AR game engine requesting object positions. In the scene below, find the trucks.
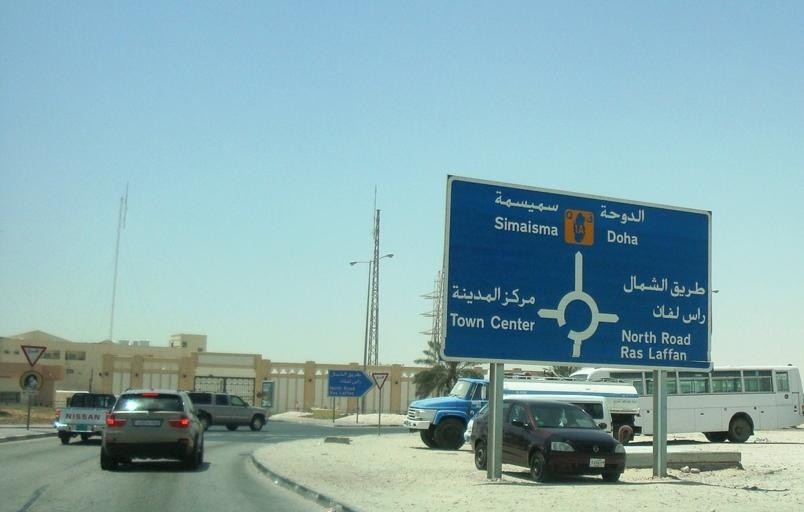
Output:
[404,378,642,450]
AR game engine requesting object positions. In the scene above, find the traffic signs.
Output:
[328,367,378,399]
[438,172,717,373]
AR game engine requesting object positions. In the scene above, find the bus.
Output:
[568,366,803,445]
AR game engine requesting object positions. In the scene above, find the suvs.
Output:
[98,386,205,472]
[186,391,269,432]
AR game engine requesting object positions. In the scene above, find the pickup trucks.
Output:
[54,389,118,447]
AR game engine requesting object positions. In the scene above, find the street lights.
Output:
[346,253,395,413]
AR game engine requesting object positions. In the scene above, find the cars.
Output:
[465,395,627,485]
